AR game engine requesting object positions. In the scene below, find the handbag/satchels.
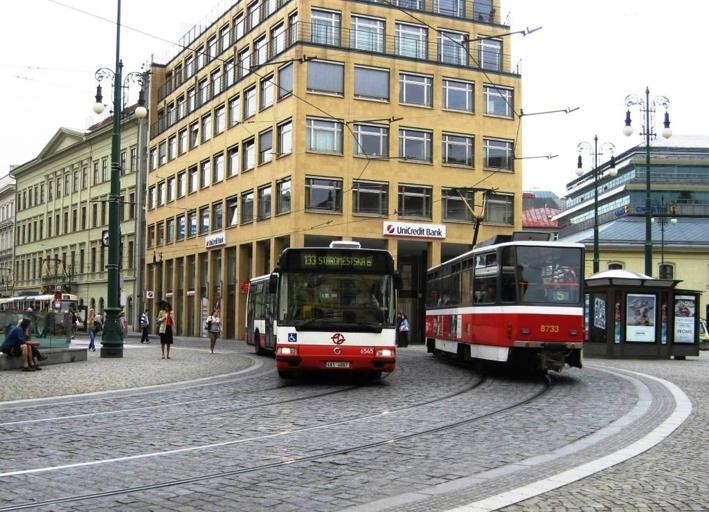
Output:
[204,314,214,331]
[399,325,409,333]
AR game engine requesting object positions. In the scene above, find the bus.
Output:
[1,293,80,338]
[423,229,584,379]
[232,230,404,382]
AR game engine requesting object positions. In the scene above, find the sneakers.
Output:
[89,346,91,351]
[93,348,96,352]
[37,355,50,361]
[141,339,152,344]
[29,365,42,370]
[161,354,166,359]
[167,355,171,359]
[209,351,216,354]
[21,366,36,372]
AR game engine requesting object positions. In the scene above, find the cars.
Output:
[699,318,709,342]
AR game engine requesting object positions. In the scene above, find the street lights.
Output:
[91,59,149,359]
[651,194,677,280]
[63,264,77,292]
[575,134,618,273]
[623,84,673,278]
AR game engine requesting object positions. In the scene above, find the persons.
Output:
[397,313,409,348]
[437,254,577,308]
[157,303,175,359]
[16,318,48,361]
[139,307,151,345]
[94,312,103,328]
[0,316,43,372]
[120,311,129,344]
[26,306,79,345]
[348,276,381,308]
[395,311,402,345]
[205,308,222,355]
[86,308,97,353]
